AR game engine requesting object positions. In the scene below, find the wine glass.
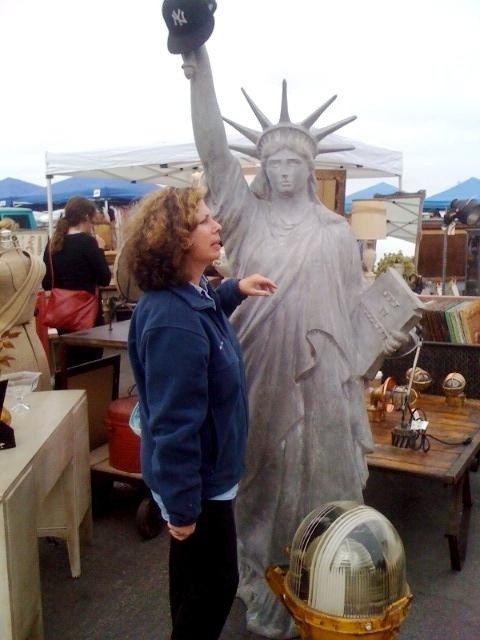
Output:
[1,370,44,417]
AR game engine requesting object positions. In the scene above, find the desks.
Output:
[364,385,480,571]
[61,319,165,538]
[0,388,93,640]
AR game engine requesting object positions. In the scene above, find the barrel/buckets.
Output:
[104,395,140,472]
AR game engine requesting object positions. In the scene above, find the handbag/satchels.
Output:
[42,287,99,330]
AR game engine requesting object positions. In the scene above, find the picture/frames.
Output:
[56,352,122,452]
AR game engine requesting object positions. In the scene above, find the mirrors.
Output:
[350,190,425,283]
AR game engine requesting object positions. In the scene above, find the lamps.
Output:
[440,198,480,295]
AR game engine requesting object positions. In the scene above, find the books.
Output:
[349,266,428,383]
[418,299,479,346]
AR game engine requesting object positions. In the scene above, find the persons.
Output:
[120,182,280,640]
[178,40,414,639]
[40,194,112,371]
